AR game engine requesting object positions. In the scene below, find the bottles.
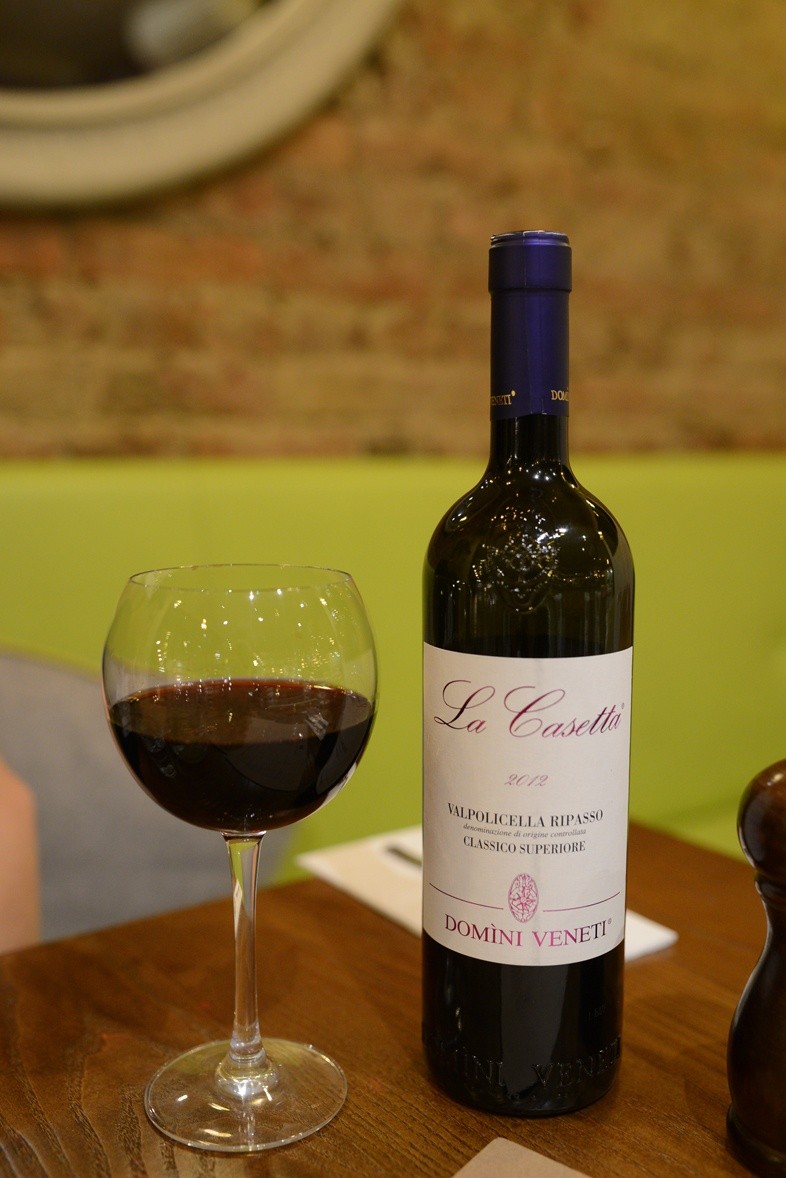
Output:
[723,759,786,1178]
[421,226,636,1121]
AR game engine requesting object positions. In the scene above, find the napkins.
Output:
[293,830,680,975]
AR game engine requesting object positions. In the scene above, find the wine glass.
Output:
[100,562,377,1153]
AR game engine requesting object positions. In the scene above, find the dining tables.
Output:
[2,822,774,1178]
